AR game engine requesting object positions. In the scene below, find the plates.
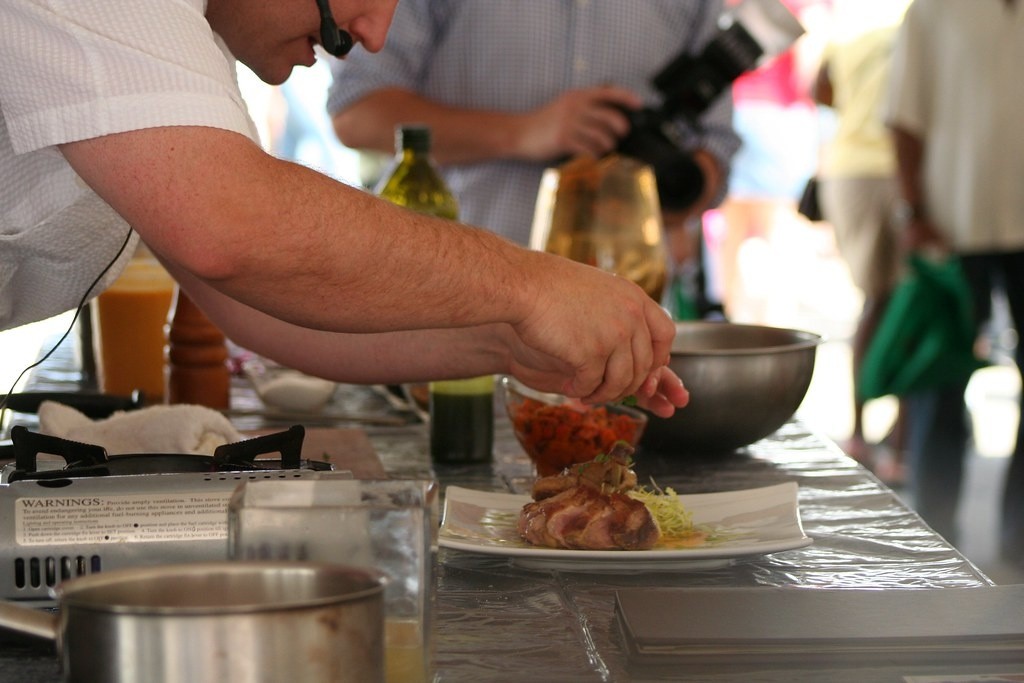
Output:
[439,481,812,571]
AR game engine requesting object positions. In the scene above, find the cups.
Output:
[431,378,496,465]
[92,236,180,394]
[534,165,667,302]
[228,469,438,682]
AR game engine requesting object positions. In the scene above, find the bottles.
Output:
[380,125,461,226]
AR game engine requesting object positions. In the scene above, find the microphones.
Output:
[316,0,353,55]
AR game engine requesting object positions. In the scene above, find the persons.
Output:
[328,0,739,324]
[0,0,690,417]
[813,0,1024,560]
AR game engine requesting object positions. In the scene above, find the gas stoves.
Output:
[0,425,376,602]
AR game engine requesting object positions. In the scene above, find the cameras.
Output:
[604,1,804,213]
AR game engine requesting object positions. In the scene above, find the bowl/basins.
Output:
[635,319,823,455]
[50,561,388,683]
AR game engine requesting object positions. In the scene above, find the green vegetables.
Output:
[579,390,639,475]
